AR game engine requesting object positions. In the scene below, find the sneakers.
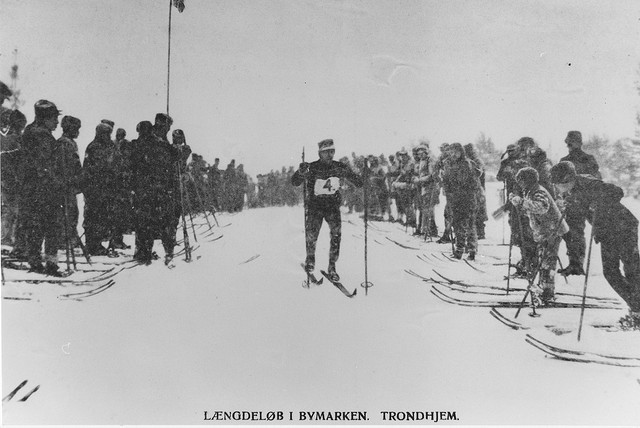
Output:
[328,266,340,282]
[536,291,556,301]
[562,263,585,275]
[305,257,315,272]
[621,316,639,329]
[29,261,45,273]
[469,251,476,259]
[454,251,463,261]
[44,263,62,277]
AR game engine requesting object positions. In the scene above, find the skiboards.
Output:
[424,269,623,301]
[0,266,125,284]
[174,212,239,245]
[343,219,426,250]
[487,307,620,333]
[300,262,357,298]
[2,279,116,300]
[57,257,141,269]
[428,286,624,307]
[419,242,512,271]
[525,332,640,366]
[110,246,200,259]
[402,269,462,284]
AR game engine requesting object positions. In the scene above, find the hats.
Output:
[550,160,576,184]
[61,115,81,129]
[35,99,62,117]
[515,166,539,185]
[10,108,26,125]
[449,142,466,154]
[416,144,429,155]
[155,113,173,125]
[95,123,112,133]
[318,137,336,152]
[564,130,582,147]
[137,120,153,131]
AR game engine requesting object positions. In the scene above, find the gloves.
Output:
[361,166,370,177]
[299,161,311,175]
[512,195,522,206]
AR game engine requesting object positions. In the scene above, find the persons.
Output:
[85,124,115,256]
[152,113,173,141]
[557,130,602,275]
[464,143,488,239]
[111,128,133,235]
[10,101,62,261]
[193,153,300,212]
[128,119,192,264]
[100,119,115,124]
[508,167,570,306]
[548,161,640,331]
[291,139,363,281]
[339,142,449,244]
[445,142,481,260]
[26,104,67,278]
[518,136,535,153]
[56,114,85,250]
[170,128,193,216]
[1,81,24,245]
[494,143,529,244]
[526,146,556,200]
